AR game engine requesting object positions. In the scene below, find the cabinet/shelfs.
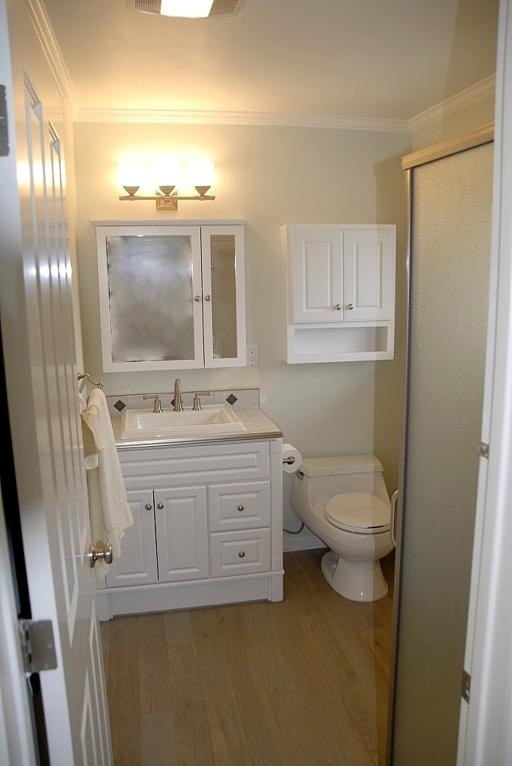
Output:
[207,479,275,579]
[96,223,246,375]
[100,485,210,589]
[280,220,400,366]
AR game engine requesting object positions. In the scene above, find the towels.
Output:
[81,388,135,582]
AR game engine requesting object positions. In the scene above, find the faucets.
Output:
[174,378,185,411]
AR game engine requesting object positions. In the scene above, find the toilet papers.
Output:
[282,441,303,475]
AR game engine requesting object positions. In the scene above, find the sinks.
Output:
[121,403,248,439]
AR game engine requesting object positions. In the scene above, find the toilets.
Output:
[293,455,395,603]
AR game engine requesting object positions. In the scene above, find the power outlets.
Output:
[246,344,259,367]
